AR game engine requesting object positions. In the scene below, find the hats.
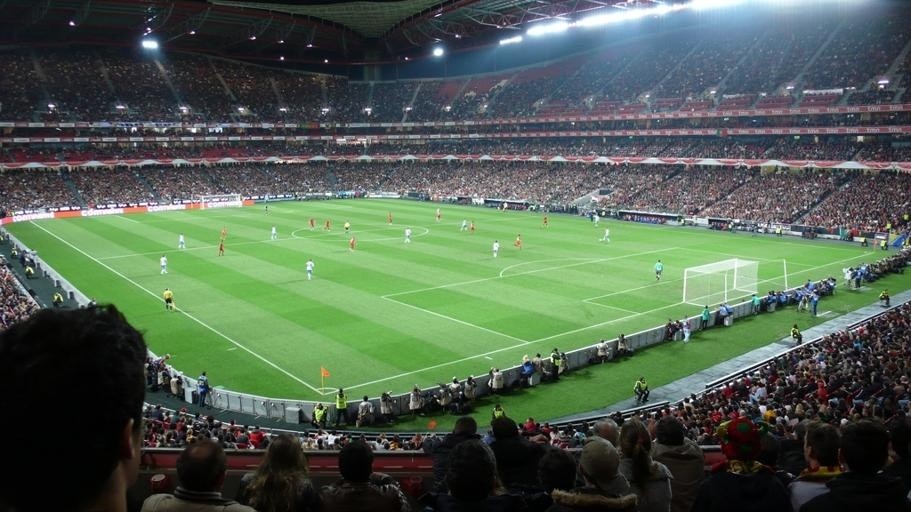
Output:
[579,438,632,497]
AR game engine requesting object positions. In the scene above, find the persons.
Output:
[0,11,910,512]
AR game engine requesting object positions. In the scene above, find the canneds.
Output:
[149,474,168,492]
